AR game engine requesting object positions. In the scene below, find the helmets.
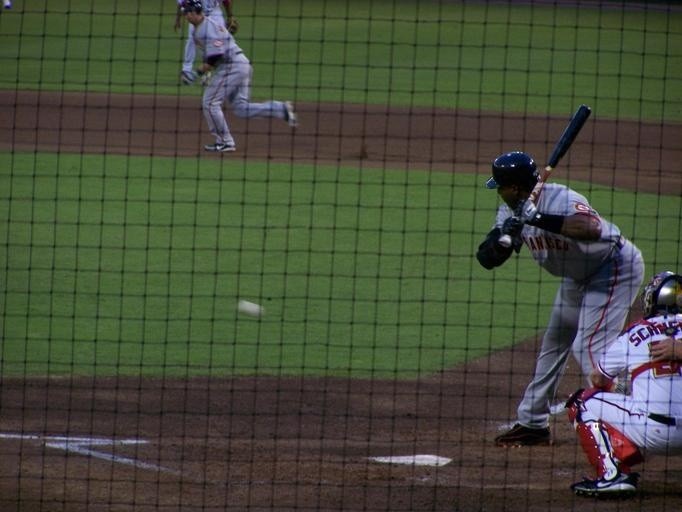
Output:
[181,0,203,16]
[641,271,682,319]
[486,152,536,189]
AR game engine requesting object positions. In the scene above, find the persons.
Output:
[649,337,682,362]
[475,149,647,441]
[3,0,11,9]
[172,0,239,83]
[565,270,682,500]
[178,0,297,152]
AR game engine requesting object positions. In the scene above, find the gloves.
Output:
[502,217,524,236]
[514,197,541,225]
[182,70,197,83]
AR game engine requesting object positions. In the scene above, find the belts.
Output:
[616,233,625,250]
[649,413,676,425]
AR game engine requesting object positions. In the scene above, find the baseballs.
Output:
[244,303,262,317]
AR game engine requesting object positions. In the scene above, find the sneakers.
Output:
[283,101,297,127]
[570,472,639,498]
[205,143,236,153]
[495,424,550,447]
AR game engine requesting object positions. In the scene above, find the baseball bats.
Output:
[499,103,590,248]
